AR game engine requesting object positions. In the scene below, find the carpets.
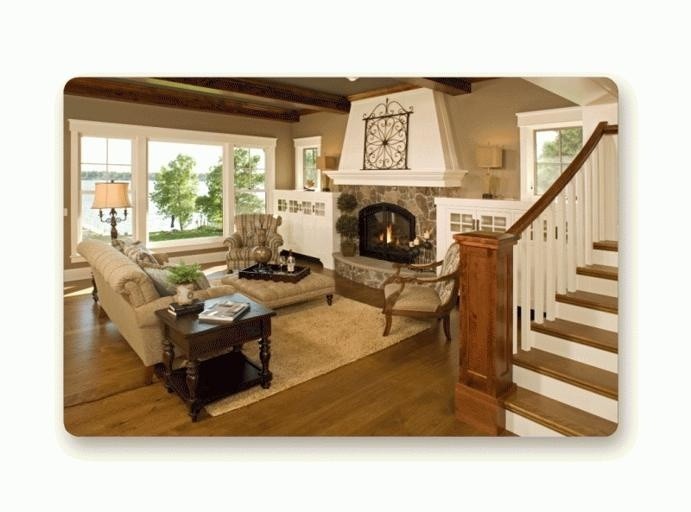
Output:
[163,262,432,419]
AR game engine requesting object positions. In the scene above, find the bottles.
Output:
[287,248,295,274]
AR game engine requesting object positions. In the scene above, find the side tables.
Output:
[152,291,277,425]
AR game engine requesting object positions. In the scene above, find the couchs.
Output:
[76,237,236,387]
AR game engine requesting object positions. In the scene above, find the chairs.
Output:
[377,242,460,341]
[222,213,285,273]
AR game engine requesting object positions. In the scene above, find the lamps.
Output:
[475,141,503,198]
[90,178,133,241]
[315,153,337,191]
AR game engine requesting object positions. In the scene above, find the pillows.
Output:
[119,242,180,297]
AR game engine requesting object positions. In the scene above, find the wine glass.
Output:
[276,255,286,275]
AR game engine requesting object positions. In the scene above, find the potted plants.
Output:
[334,193,361,258]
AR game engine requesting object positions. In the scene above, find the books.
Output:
[167,298,204,317]
[198,299,251,321]
